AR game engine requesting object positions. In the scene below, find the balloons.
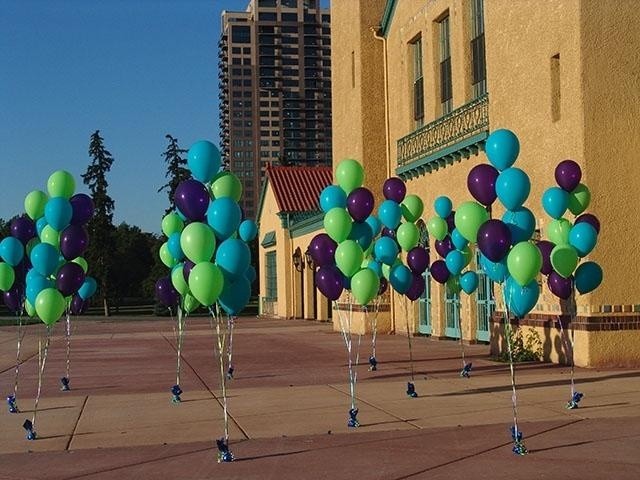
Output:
[309,127,605,318]
[155,141,257,316]
[1,171,97,327]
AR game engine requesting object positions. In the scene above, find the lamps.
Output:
[292,246,306,273]
[304,245,316,271]
